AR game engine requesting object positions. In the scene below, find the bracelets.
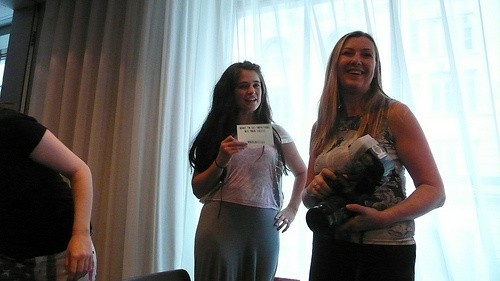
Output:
[214,159,226,170]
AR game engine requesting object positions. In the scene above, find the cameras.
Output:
[305,134,395,235]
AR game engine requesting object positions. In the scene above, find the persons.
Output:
[0,100,97,281]
[189,61,307,281]
[302,31,445,281]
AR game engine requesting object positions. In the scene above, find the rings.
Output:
[314,183,318,190]
